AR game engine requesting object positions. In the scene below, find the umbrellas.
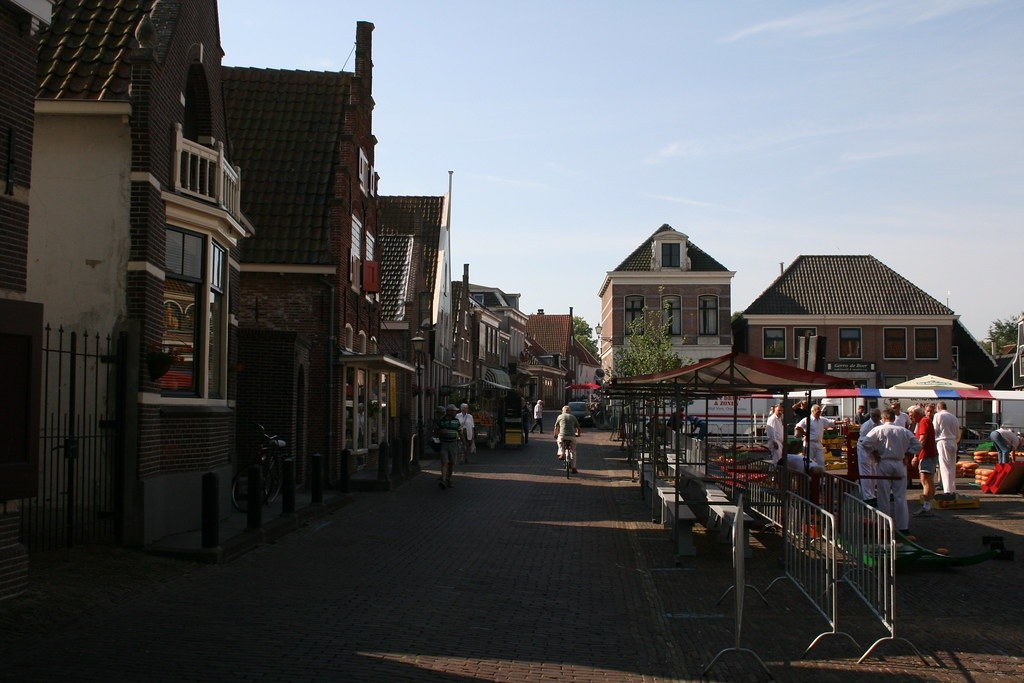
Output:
[568,383,601,398]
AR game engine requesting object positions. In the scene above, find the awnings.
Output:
[482,368,513,391]
[341,349,415,374]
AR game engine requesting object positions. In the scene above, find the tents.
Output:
[889,373,978,427]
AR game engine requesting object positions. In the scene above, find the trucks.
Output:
[819,397,967,426]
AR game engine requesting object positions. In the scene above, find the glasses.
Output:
[872,415,881,418]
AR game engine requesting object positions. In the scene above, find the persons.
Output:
[687,415,706,440]
[667,407,686,432]
[455,403,475,462]
[863,408,922,534]
[792,399,808,423]
[765,403,784,476]
[437,404,463,488]
[524,401,533,426]
[990,428,1024,464]
[529,400,545,434]
[794,404,848,485]
[857,402,960,516]
[553,405,581,474]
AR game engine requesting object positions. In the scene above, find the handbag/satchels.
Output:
[427,431,441,452]
[470,440,476,455]
[449,440,466,464]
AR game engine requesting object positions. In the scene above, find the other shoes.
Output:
[529,430,535,434]
[924,501,931,511]
[572,468,578,474]
[559,454,565,459]
[540,431,544,434]
[438,477,456,489]
[899,529,910,534]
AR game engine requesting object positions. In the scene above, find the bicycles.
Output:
[232,425,293,513]
[556,435,580,478]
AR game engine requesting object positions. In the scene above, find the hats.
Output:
[446,404,459,410]
[537,399,543,404]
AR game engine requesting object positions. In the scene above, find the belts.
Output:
[859,440,862,442]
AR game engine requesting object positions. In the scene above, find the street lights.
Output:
[412,330,426,456]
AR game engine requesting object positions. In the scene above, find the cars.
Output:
[568,403,595,426]
